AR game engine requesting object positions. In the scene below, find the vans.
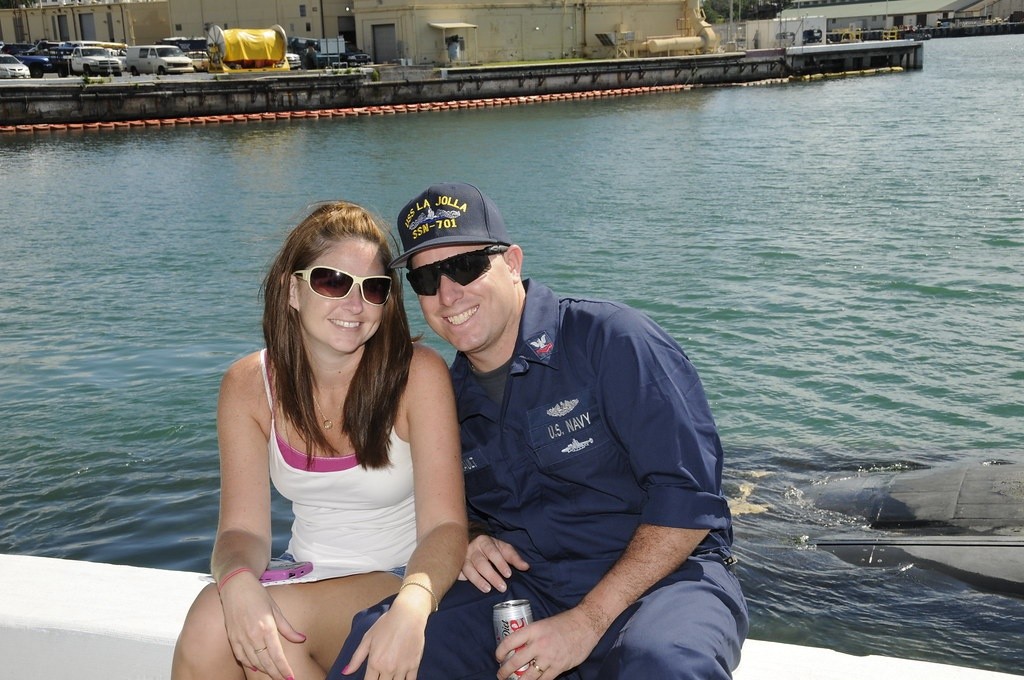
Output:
[126,45,194,76]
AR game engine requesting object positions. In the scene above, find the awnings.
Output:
[429,21,478,65]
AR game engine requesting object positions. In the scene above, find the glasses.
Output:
[290,264,395,307]
[405,247,507,296]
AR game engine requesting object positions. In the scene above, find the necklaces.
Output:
[313,395,344,429]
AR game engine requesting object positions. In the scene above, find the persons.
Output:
[325,182,749,680]
[752,30,759,49]
[172,200,468,680]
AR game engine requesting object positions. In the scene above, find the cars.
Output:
[314,42,371,69]
[184,51,210,72]
[286,53,302,70]
[0,54,31,80]
[14,56,51,78]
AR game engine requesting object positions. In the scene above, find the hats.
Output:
[390,178,512,269]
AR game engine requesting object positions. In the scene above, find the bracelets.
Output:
[218,567,255,593]
[400,581,438,611]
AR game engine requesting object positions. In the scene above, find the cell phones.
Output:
[260,561,313,583]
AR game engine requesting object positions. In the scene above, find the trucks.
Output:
[52,46,123,78]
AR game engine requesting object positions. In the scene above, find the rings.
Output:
[533,662,544,674]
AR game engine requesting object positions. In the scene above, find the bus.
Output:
[63,41,126,56]
[0,44,35,55]
[33,40,64,54]
[153,37,208,51]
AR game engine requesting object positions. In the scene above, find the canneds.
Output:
[492,599,535,680]
